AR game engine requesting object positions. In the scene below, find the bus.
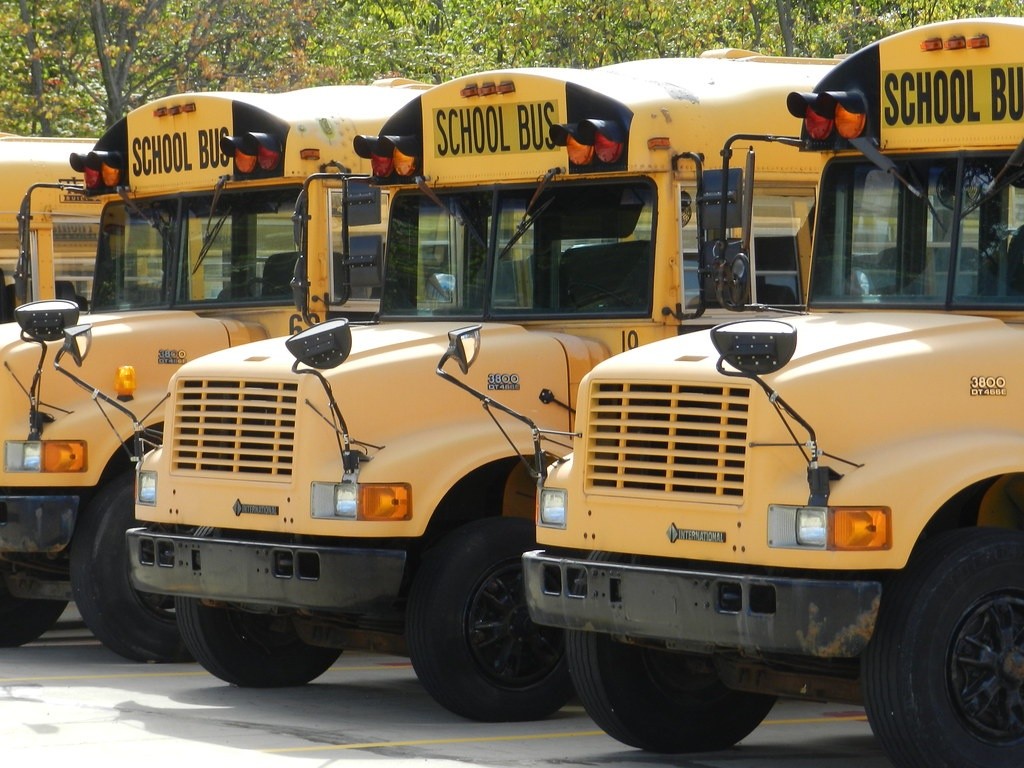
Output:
[125,43,845,724]
[0,75,512,670]
[517,18,1024,767]
[1,127,207,316]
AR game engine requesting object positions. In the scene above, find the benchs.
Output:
[872,246,980,298]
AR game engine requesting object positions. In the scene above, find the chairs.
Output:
[1,280,16,323]
[1008,225,1024,301]
[562,241,652,318]
[260,252,346,304]
[55,280,87,311]
